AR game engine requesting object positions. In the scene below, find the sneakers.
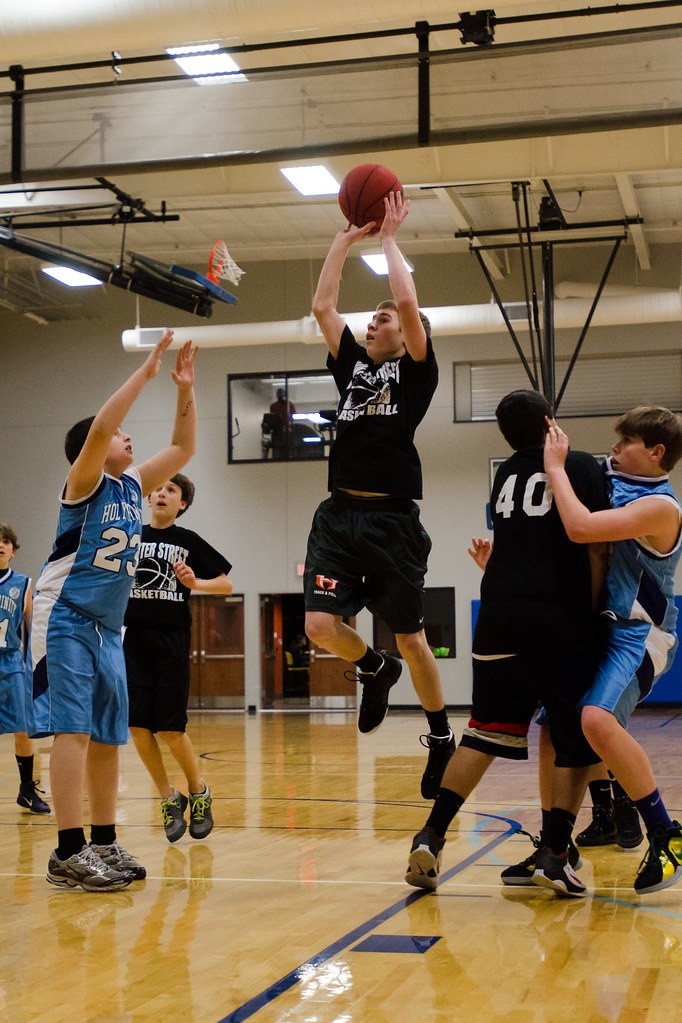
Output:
[161,787,189,844]
[188,779,214,839]
[418,728,456,799]
[633,820,682,895]
[17,780,51,814]
[45,846,133,892]
[531,848,587,897]
[501,837,583,884]
[403,828,447,891]
[343,653,403,735]
[575,798,644,848]
[89,839,147,880]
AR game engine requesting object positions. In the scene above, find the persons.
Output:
[118,472,234,844]
[406,389,682,904]
[26,331,199,892]
[269,386,296,459]
[303,191,458,799]
[0,521,52,814]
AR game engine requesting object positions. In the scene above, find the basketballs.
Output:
[337,162,404,232]
